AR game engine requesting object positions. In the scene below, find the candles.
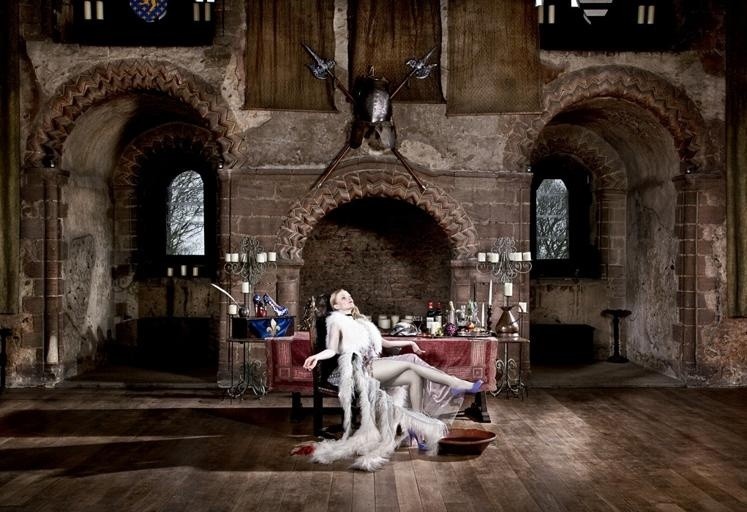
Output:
[241,281,249,293]
[477,251,532,264]
[504,282,513,296]
[226,250,276,264]
[166,264,199,276]
[228,304,237,314]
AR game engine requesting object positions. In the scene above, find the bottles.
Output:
[426,301,455,334]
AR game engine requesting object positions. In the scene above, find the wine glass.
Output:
[413,316,422,334]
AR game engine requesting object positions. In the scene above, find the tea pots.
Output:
[496,305,523,334]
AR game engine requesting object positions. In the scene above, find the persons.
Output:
[291,286,484,473]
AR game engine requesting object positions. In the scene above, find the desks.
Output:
[256,330,498,424]
[496,336,533,402]
[225,335,270,402]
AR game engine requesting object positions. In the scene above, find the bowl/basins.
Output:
[434,428,497,455]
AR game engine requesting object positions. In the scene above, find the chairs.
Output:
[311,310,363,440]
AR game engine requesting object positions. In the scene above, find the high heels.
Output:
[451,380,484,397]
[253,294,262,317]
[408,428,433,451]
[263,293,288,316]
